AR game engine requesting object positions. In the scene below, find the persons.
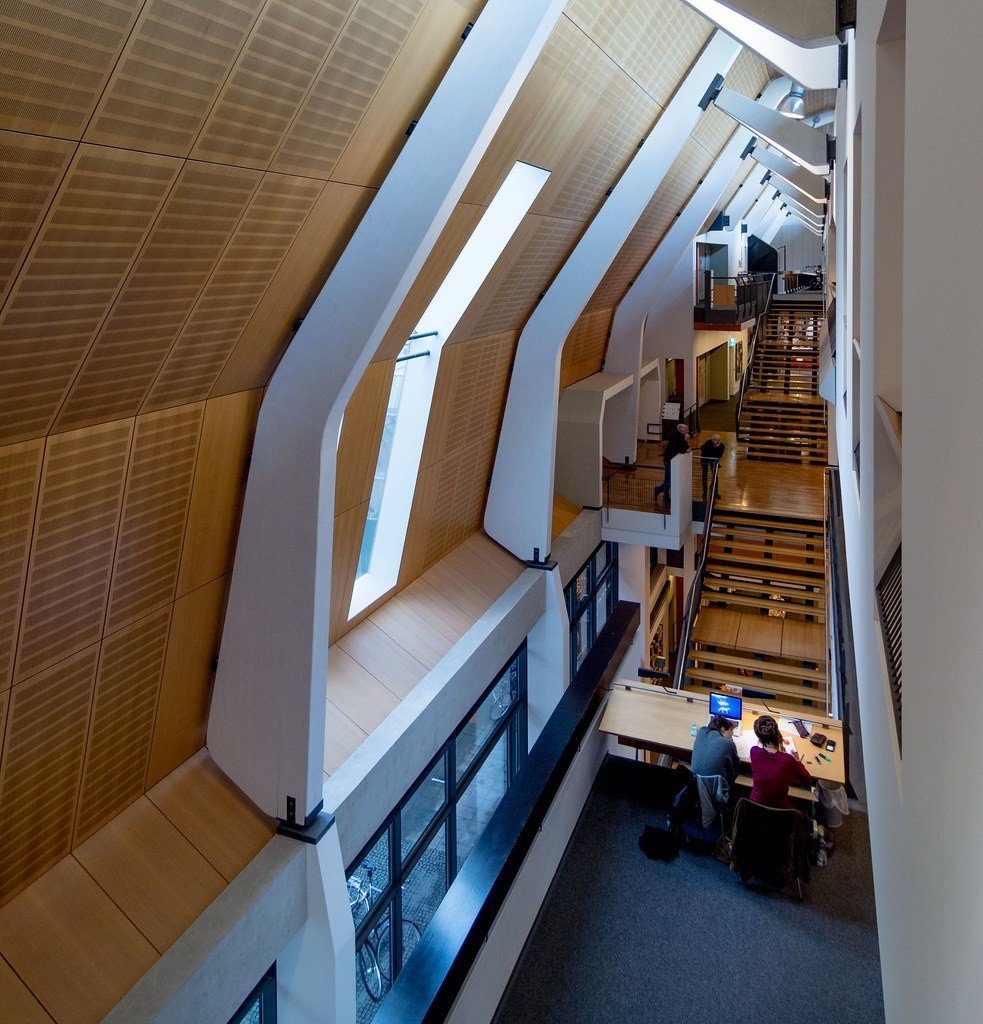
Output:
[654,424,691,508]
[806,323,813,341]
[700,434,726,503]
[691,715,742,811]
[750,715,813,845]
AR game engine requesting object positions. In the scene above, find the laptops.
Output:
[708,692,742,737]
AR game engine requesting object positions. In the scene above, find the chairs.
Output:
[667,766,731,860]
[728,797,814,891]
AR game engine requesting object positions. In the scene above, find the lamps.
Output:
[779,87,806,120]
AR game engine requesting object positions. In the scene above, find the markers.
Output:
[815,756,820,763]
[818,752,830,761]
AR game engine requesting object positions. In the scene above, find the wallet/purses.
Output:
[810,733,826,747]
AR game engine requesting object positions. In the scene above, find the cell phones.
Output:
[826,740,835,752]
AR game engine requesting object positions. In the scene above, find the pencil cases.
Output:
[788,721,810,738]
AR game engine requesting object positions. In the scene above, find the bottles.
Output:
[691,723,698,736]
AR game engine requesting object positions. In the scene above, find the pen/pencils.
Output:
[800,754,804,761]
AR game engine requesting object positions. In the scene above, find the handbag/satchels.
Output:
[639,824,679,862]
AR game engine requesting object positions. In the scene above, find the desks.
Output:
[598,676,846,785]
